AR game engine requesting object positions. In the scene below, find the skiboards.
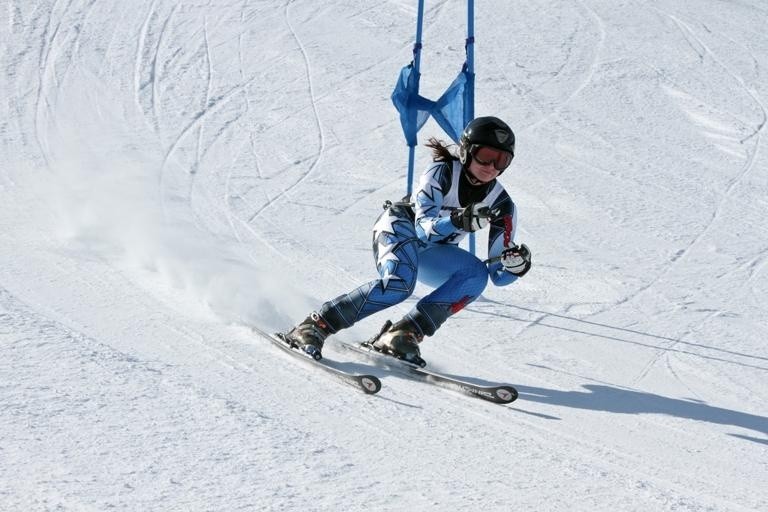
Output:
[251,328,518,404]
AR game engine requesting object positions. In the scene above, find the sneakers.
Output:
[286,310,330,356]
[373,319,424,365]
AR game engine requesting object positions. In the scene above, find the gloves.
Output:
[500,241,531,277]
[450,202,491,232]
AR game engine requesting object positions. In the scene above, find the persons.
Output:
[286,116,532,366]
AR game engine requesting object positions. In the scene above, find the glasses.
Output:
[468,143,514,170]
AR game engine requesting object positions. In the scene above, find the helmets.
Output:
[463,117,514,155]
